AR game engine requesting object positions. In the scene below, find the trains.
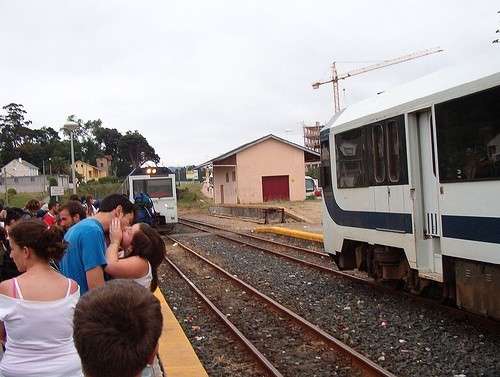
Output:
[116,159,178,233]
[322,48,500,325]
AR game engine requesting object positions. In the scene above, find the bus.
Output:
[305,176,317,200]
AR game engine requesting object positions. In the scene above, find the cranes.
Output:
[311,45,444,114]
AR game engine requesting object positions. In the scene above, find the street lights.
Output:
[42,158,52,193]
[64,120,79,194]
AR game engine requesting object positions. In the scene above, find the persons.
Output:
[70,194,79,201]
[84,194,99,218]
[0,202,22,283]
[81,196,86,204]
[23,198,41,219]
[72,278,164,377]
[58,193,136,297]
[104,218,166,377]
[60,201,86,231]
[42,199,59,227]
[93,198,102,208]
[0,218,85,377]
